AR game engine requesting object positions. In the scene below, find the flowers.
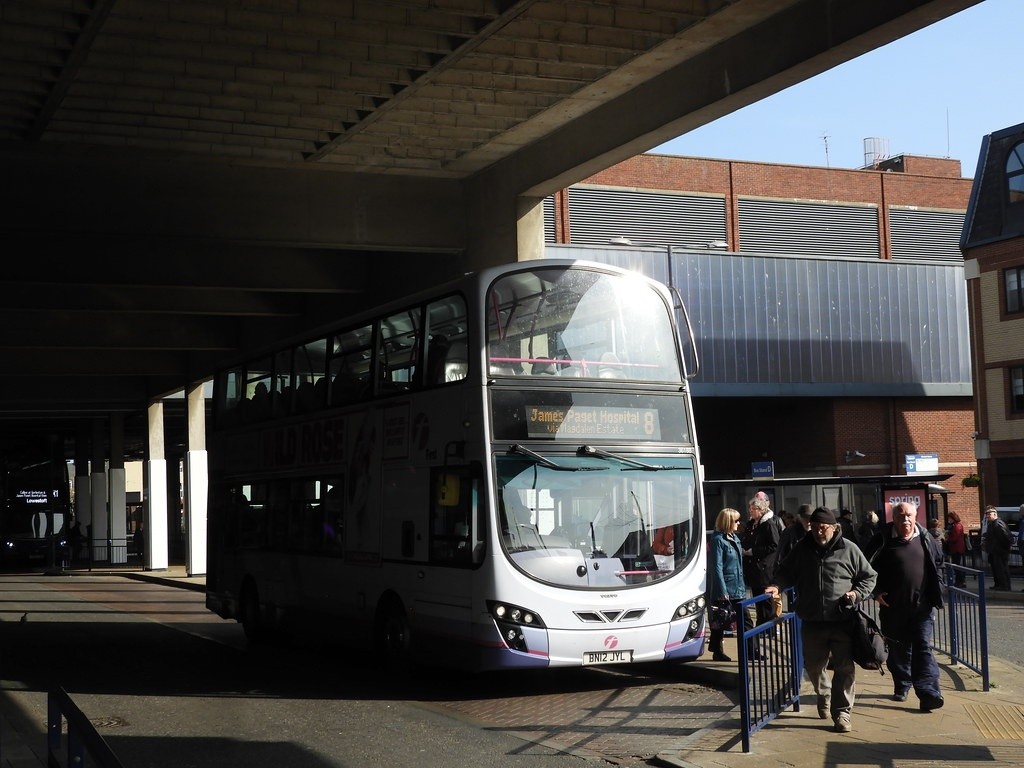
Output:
[961,474,980,484]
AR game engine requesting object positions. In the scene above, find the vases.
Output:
[965,481,977,487]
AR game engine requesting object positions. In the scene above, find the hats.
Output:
[840,510,853,517]
[797,504,815,517]
[984,505,997,513]
[808,506,837,524]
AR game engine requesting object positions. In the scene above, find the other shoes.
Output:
[832,716,851,731]
[920,695,944,712]
[748,650,769,661]
[817,696,831,719]
[956,583,966,588]
[989,582,999,589]
[713,653,731,662]
[994,585,1011,591]
[759,633,769,638]
[893,689,908,702]
[953,582,958,586]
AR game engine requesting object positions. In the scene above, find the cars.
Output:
[980,506,1023,569]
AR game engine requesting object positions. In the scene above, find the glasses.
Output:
[748,507,758,512]
[735,518,741,523]
[810,525,831,533]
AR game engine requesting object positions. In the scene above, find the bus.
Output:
[196,259,711,672]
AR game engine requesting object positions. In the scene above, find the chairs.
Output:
[221,352,625,429]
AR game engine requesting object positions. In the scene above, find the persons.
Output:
[1016,504,1024,592]
[928,518,943,538]
[773,503,813,572]
[741,491,780,638]
[134,521,144,559]
[707,507,769,662]
[767,505,878,732]
[865,502,945,710]
[70,521,82,558]
[946,511,967,588]
[252,382,270,400]
[984,504,1014,591]
[835,509,856,544]
[859,511,879,545]
[652,514,674,555]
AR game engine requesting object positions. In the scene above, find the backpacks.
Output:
[840,592,890,670]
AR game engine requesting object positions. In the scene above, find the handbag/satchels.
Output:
[708,598,737,631]
[964,534,972,550]
